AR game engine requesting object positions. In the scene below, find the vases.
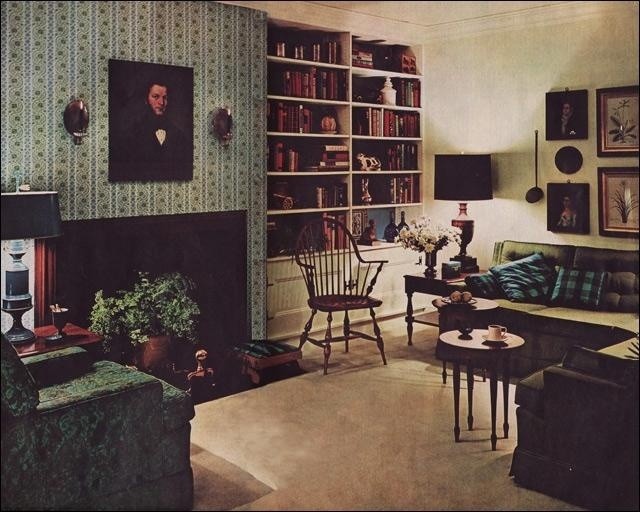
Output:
[421,244,439,277]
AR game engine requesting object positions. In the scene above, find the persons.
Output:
[558,103,586,137]
[124,82,182,178]
[557,195,578,228]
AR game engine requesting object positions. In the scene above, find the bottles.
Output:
[379,76,398,106]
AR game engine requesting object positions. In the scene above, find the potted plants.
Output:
[79,256,214,389]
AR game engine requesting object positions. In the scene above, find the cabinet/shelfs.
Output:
[258,14,436,344]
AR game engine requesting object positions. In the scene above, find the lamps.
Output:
[0,176,68,351]
[210,105,238,151]
[429,149,496,275]
[62,96,92,148]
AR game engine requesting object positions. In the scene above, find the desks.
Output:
[432,327,529,453]
[427,292,500,389]
[8,319,105,366]
[398,264,491,348]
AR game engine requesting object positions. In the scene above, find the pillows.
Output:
[546,260,610,311]
[463,269,508,303]
[488,247,558,304]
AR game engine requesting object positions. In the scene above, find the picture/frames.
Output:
[594,84,640,159]
[595,165,640,240]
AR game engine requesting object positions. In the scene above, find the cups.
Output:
[488,325,507,340]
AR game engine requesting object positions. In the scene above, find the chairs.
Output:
[291,214,393,377]
[0,326,205,511]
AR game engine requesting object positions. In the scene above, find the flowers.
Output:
[388,212,467,258]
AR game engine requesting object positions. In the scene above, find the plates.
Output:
[554,145,584,175]
[481,334,509,343]
[441,298,477,308]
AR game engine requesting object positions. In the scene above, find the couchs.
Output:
[503,332,640,510]
[430,237,638,380]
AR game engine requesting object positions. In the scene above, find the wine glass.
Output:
[52,308,68,338]
[455,319,475,340]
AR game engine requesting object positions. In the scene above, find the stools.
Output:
[223,336,312,388]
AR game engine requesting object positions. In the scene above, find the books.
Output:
[366,81,420,138]
[276,42,285,57]
[317,144,349,171]
[268,144,299,172]
[267,67,343,133]
[387,144,417,169]
[390,173,418,203]
[313,44,320,62]
[322,213,347,249]
[329,40,337,63]
[295,45,304,59]
